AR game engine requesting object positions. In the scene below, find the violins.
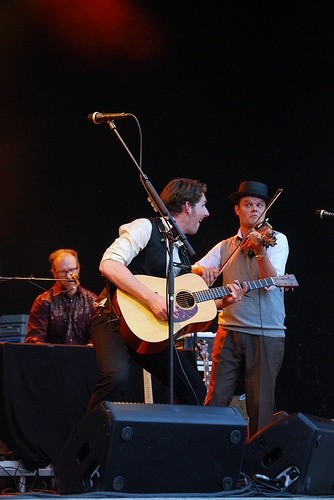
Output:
[241,223,277,259]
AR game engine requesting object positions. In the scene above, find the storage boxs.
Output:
[176,331,219,373]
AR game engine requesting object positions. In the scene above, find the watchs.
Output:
[255,253,266,261]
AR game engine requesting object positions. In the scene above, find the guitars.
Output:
[112,273,299,354]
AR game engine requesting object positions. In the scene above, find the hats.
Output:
[231,181,271,206]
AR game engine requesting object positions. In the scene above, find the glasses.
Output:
[52,263,79,276]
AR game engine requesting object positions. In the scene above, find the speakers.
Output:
[241,412,334,494]
[53,401,248,494]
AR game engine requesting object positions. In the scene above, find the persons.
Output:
[55,177,248,495]
[25,249,98,345]
[189,182,290,438]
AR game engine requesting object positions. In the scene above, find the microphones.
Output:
[315,209,334,219]
[72,274,80,286]
[88,112,132,125]
